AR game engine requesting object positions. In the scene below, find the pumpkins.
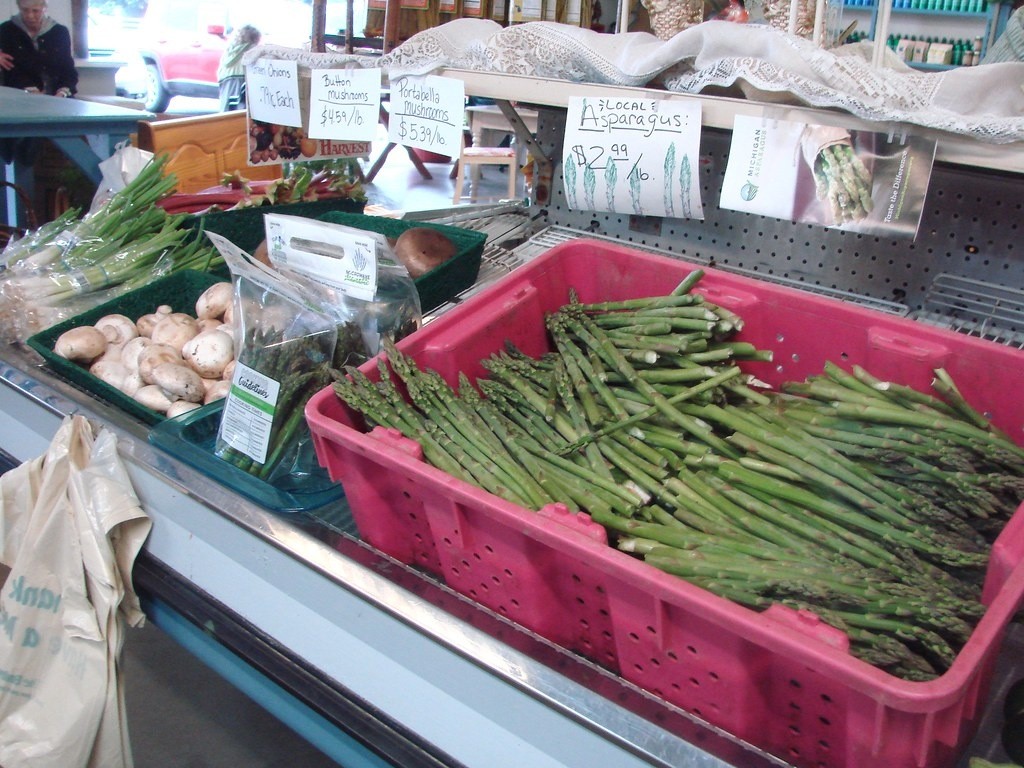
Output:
[257,131,316,157]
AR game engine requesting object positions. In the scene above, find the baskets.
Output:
[303,236,1024,768]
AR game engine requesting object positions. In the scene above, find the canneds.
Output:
[961,36,983,67]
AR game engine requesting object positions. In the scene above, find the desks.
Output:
[0,85,157,191]
[466,104,541,179]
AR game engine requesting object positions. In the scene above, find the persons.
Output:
[217,24,261,112]
[0,0,93,227]
[978,0,1024,65]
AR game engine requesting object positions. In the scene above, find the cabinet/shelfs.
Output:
[839,0,1002,78]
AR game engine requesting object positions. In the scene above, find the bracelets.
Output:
[59,87,71,98]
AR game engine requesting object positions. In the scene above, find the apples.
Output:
[250,124,280,164]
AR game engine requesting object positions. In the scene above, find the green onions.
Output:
[0,147,227,347]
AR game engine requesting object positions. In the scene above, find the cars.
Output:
[116,0,382,114]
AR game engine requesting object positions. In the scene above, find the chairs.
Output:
[454,129,517,203]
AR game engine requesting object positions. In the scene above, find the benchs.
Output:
[142,48,223,113]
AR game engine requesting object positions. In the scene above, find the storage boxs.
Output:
[25,268,264,419]
[303,239,1024,768]
[304,210,487,311]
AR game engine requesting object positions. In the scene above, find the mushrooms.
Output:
[52,282,291,420]
[250,227,458,281]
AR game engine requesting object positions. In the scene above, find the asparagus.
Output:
[216,302,419,483]
[323,270,1024,686]
[812,144,874,227]
